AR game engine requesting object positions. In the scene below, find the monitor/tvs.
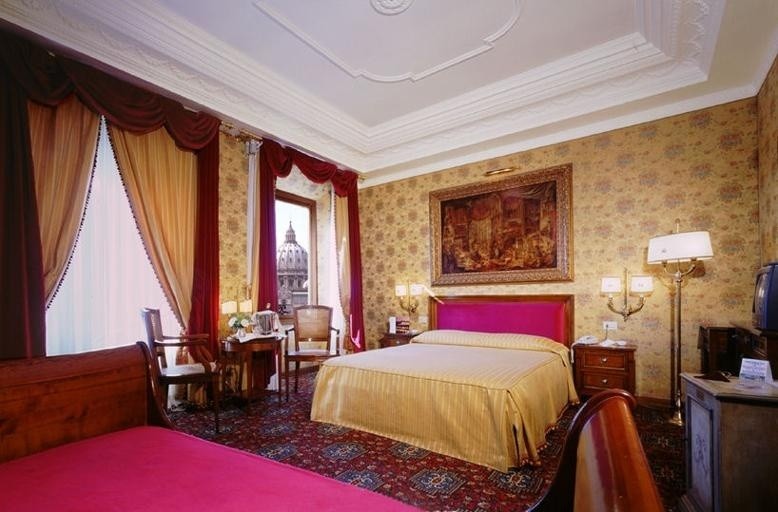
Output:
[752,263,778,334]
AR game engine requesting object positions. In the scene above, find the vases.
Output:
[236,328,247,338]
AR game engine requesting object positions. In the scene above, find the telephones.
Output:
[578,335,597,344]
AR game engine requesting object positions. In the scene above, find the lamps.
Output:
[600,268,654,323]
[394,280,423,322]
[646,218,715,428]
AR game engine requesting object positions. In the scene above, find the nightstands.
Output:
[378,332,419,348]
[572,341,638,410]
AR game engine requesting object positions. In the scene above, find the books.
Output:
[395,317,409,333]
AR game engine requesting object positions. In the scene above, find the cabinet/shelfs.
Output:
[675,371,778,511]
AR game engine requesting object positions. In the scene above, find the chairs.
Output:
[139,307,223,433]
[283,304,341,402]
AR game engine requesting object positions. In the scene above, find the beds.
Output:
[315,293,576,475]
[0,340,668,511]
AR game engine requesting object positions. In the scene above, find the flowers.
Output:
[228,311,253,331]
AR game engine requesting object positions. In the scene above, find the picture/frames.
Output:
[428,162,575,287]
